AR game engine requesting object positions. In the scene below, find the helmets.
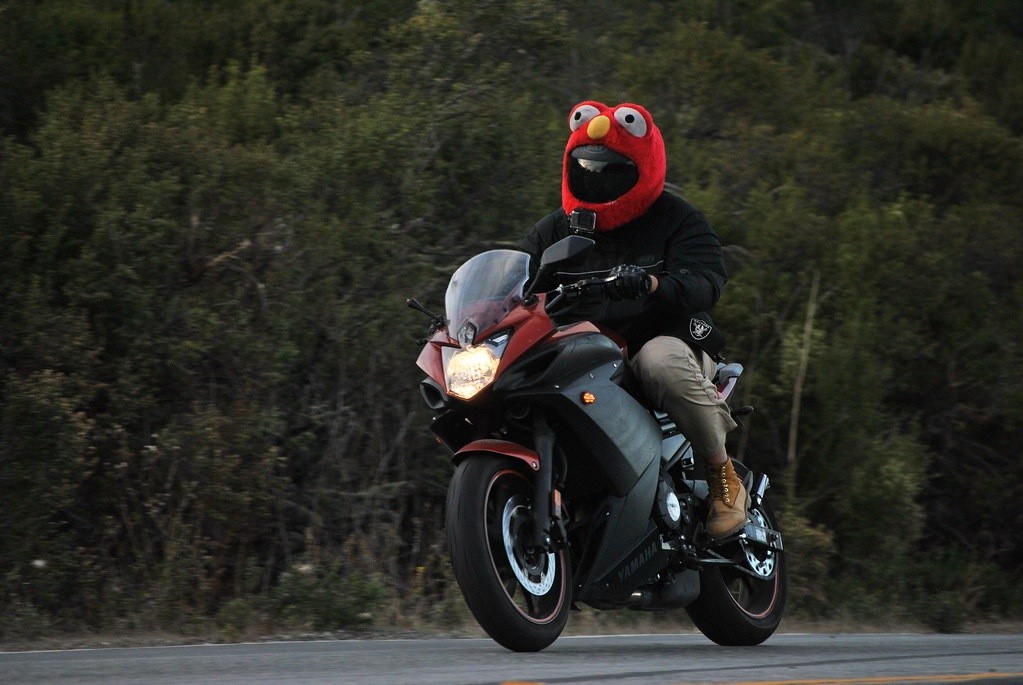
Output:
[566,144,640,203]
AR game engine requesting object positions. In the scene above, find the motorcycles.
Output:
[406,236,786,652]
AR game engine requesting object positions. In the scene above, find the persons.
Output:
[487,100,752,541]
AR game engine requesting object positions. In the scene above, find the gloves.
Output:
[609,264,652,302]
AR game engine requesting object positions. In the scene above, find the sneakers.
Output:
[705,457,752,538]
